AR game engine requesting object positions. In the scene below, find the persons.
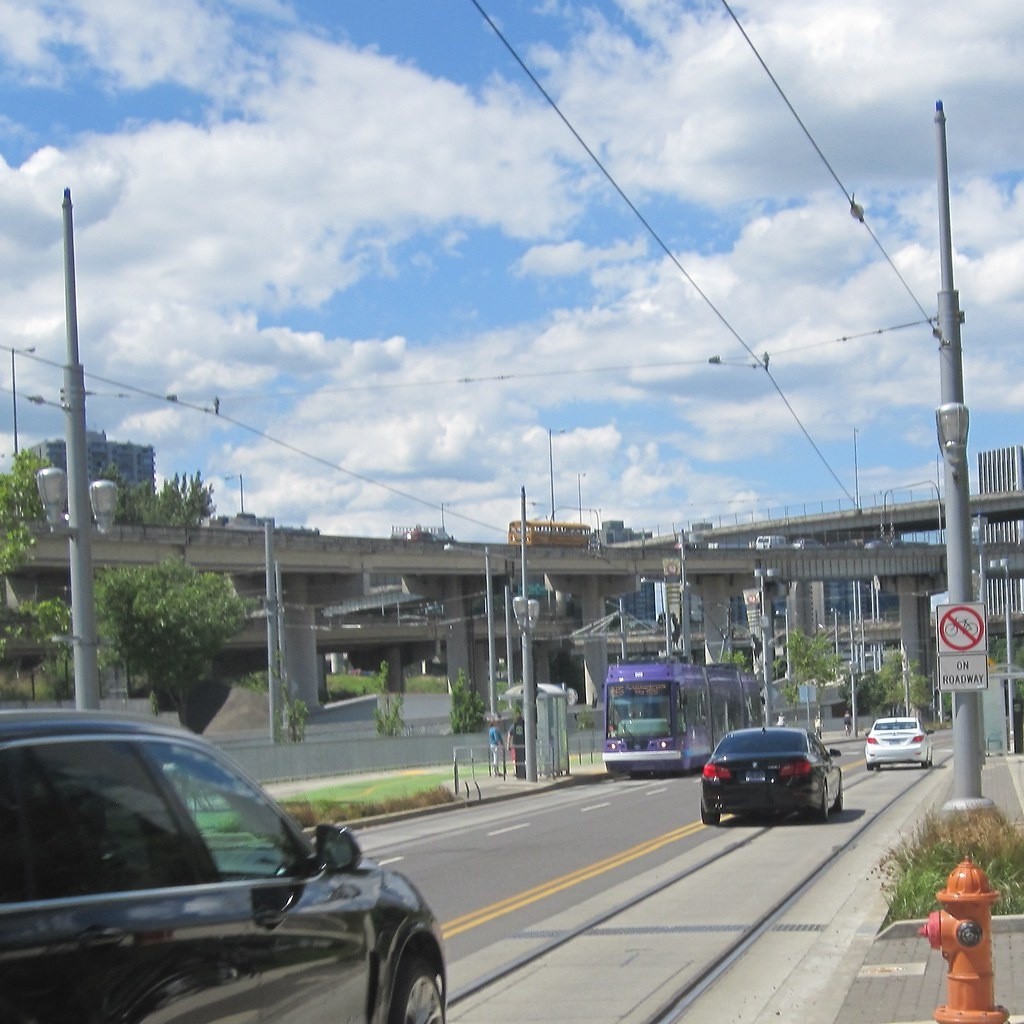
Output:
[776,712,787,727]
[507,715,524,775]
[488,722,504,776]
[678,529,688,543]
[844,710,851,732]
[814,712,824,737]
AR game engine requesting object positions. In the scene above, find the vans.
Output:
[755,534,787,550]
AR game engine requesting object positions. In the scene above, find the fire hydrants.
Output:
[916,849,1010,1024]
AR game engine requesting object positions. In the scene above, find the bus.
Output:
[507,519,591,547]
[600,663,766,779]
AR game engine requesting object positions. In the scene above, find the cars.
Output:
[0,704,448,1023]
[700,725,845,827]
[864,716,935,772]
[864,539,889,550]
[792,538,824,550]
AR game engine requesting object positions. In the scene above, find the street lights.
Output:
[511,595,541,783]
[751,567,781,727]
[676,528,705,665]
[443,542,499,721]
[548,426,566,521]
[989,557,1016,754]
[577,472,586,524]
